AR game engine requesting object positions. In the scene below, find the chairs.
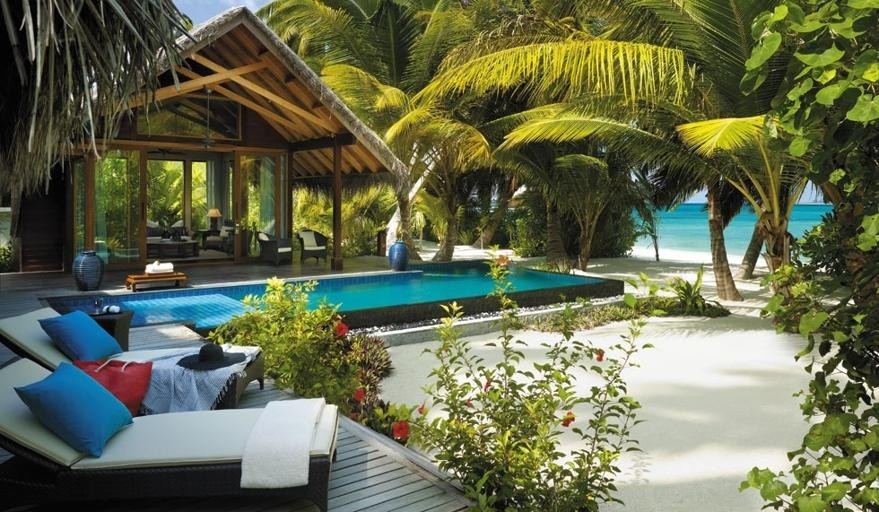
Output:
[0,306,265,415]
[0,356,340,512]
[255,230,293,267]
[297,229,328,263]
[201,219,235,256]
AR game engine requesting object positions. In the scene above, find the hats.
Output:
[175,343,247,371]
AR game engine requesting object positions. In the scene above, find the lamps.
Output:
[198,88,216,149]
[206,207,222,231]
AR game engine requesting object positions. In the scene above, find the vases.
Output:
[388,240,409,272]
[72,250,104,290]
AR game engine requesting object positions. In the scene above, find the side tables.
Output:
[198,228,219,250]
[88,311,135,352]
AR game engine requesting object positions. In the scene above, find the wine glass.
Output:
[91,297,102,315]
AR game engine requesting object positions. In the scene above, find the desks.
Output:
[146,239,201,259]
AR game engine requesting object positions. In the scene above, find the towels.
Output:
[240,398,325,490]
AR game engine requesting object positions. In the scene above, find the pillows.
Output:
[219,226,232,237]
[36,310,124,363]
[14,362,136,456]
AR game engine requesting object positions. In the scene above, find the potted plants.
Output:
[157,209,183,239]
